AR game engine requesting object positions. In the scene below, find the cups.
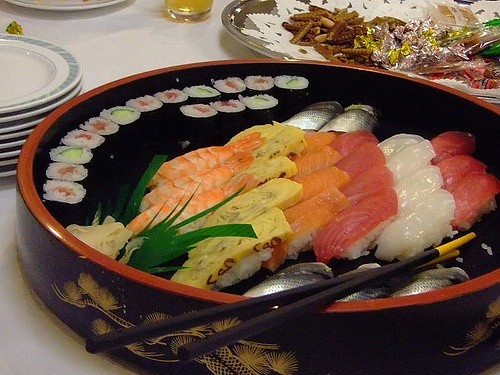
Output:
[165,0,213,23]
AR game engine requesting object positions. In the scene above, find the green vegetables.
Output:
[89,154,259,273]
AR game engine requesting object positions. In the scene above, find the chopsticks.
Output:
[83,232,476,359]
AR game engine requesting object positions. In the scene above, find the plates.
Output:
[0,0,131,11]
[0,36,86,179]
[221,0,500,104]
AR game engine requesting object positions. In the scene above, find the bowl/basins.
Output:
[15,58,500,375]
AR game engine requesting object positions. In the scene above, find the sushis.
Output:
[42,73,310,226]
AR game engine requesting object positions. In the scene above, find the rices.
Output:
[217,195,498,289]
